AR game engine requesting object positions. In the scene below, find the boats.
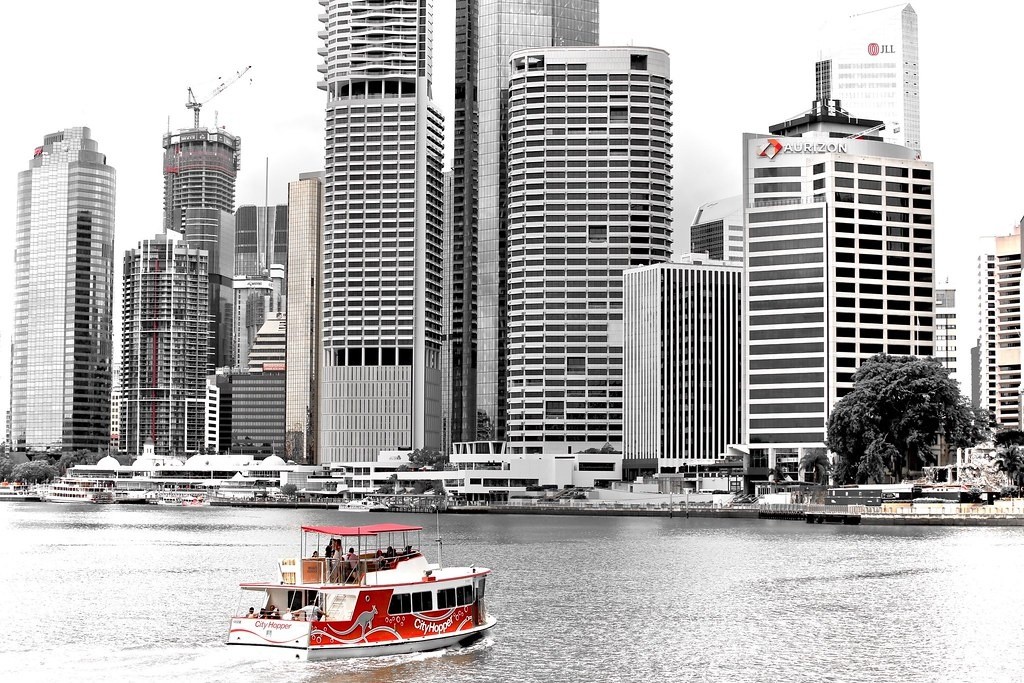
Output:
[365,492,462,514]
[1,472,273,505]
[223,522,497,667]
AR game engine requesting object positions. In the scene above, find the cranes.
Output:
[184,65,252,130]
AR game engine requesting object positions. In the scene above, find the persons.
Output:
[246,600,329,622]
[311,538,412,582]
